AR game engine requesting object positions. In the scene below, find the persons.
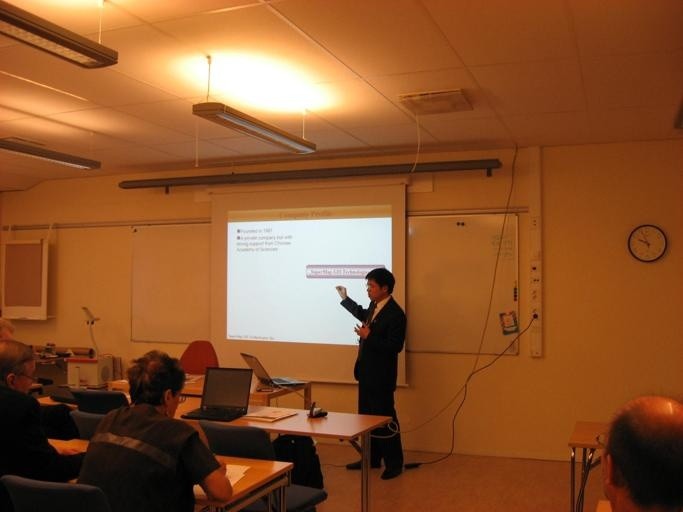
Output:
[595,395,682,512]
[335,268,403,480]
[0,339,85,484]
[0,308,15,341]
[76,349,233,511]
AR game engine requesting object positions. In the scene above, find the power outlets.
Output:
[530,303,540,321]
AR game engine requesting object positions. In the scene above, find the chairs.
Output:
[196,424,328,510]
[72,386,138,441]
[177,336,218,374]
[0,471,112,512]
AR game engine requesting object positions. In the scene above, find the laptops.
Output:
[181,367,253,422]
[240,353,305,386]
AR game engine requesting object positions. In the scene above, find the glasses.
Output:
[596,431,610,449]
[173,393,186,403]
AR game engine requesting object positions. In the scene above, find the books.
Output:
[243,406,296,423]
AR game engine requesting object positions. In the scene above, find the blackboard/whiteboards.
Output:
[130,213,520,355]
[1,239,48,322]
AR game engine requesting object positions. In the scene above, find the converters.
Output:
[404,463,419,469]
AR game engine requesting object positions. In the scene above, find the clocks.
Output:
[624,221,670,262]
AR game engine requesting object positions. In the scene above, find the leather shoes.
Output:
[382,466,402,479]
[347,458,380,470]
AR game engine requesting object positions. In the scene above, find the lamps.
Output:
[0,127,104,172]
[188,48,316,158]
[0,0,118,69]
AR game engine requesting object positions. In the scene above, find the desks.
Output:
[43,437,293,512]
[566,416,614,511]
[31,392,393,511]
[109,374,312,412]
